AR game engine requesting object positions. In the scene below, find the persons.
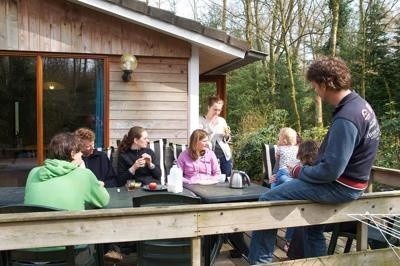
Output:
[177,128,222,185]
[76,128,124,262]
[117,126,161,188]
[198,96,233,182]
[244,57,381,266]
[16,133,110,266]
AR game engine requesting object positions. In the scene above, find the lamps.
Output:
[120,54,138,82]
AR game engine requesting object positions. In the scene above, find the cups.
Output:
[127,180,135,191]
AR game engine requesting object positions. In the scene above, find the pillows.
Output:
[168,142,189,161]
[96,146,114,162]
[261,142,278,179]
[116,138,167,185]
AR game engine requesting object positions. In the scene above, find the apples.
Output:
[149,183,157,189]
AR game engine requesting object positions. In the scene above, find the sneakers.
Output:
[104,250,123,263]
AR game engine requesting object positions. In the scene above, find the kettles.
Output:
[229,171,251,189]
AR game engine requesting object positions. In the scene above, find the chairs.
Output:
[132,193,212,266]
[324,222,359,256]
[368,217,400,255]
[261,161,277,188]
[0,204,102,266]
[164,147,176,175]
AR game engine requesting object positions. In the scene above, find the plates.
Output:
[142,185,167,191]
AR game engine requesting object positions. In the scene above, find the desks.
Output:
[184,180,272,266]
[0,186,25,208]
[99,185,196,265]
